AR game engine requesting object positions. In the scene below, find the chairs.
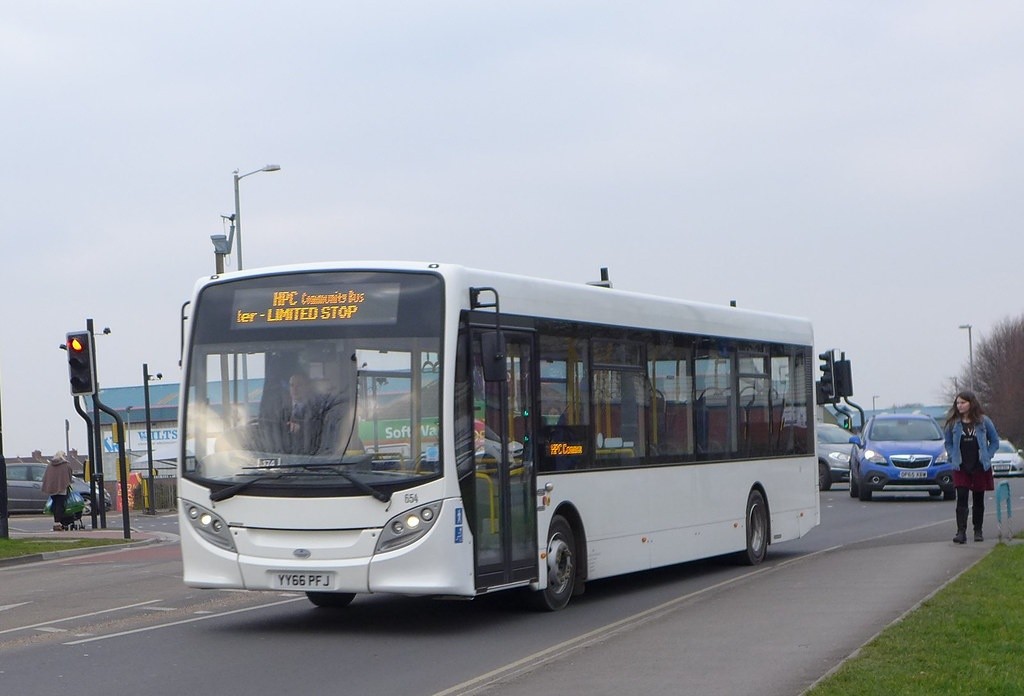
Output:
[556,387,785,460]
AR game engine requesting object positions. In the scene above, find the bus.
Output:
[174,260,819,612]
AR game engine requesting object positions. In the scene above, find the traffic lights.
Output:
[844,419,852,430]
[818,350,835,400]
[64,331,94,396]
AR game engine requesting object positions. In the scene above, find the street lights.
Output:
[872,396,878,412]
[126,406,134,462]
[235,163,281,423]
[960,325,972,393]
[89,328,112,527]
[143,374,163,515]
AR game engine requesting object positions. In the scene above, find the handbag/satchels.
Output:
[43,497,53,516]
[65,485,85,515]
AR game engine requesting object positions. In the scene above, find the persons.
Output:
[944,391,1000,544]
[273,372,323,434]
[41,451,73,531]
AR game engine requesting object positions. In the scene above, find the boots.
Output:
[972,505,985,542]
[953,507,970,543]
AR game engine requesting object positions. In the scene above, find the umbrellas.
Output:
[130,437,216,463]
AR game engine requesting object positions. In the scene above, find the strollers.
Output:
[62,485,85,531]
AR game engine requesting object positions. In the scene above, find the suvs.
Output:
[849,412,957,502]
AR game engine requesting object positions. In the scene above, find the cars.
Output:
[6,463,110,517]
[987,440,1024,477]
[815,424,856,490]
